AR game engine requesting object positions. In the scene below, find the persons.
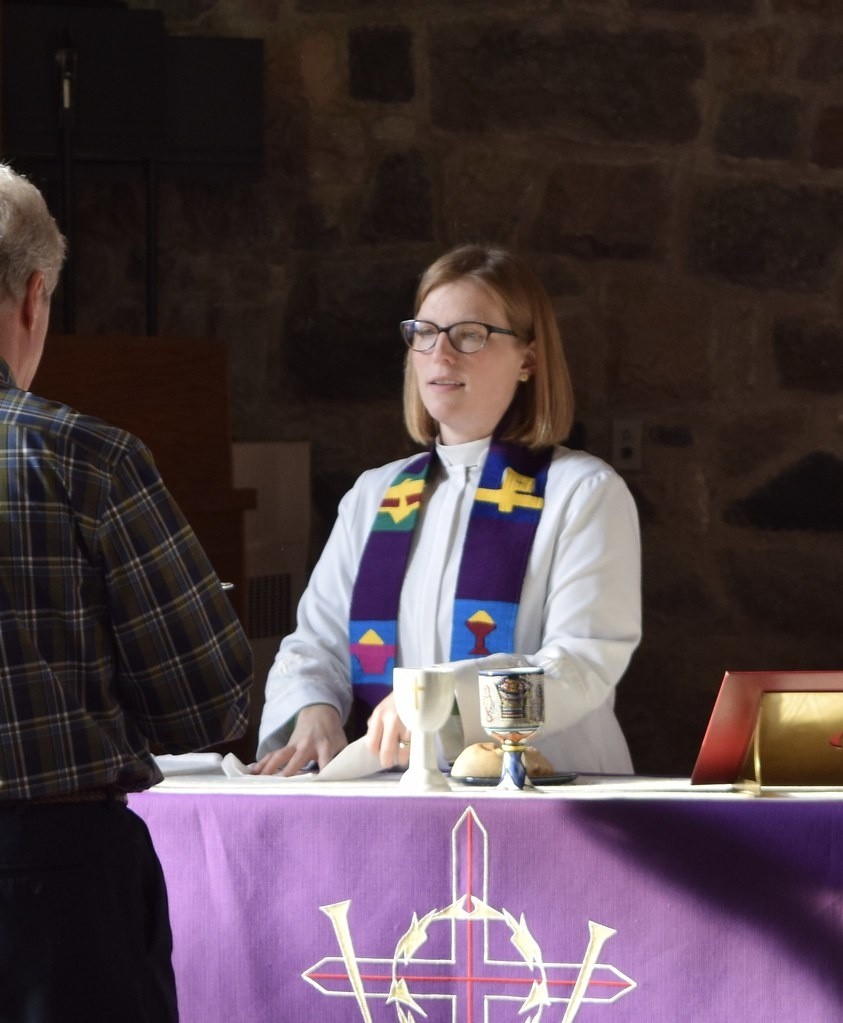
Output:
[245,246,642,777]
[1,160,255,1022]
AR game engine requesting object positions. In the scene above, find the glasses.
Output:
[401,320,519,354]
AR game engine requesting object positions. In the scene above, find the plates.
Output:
[448,773,577,787]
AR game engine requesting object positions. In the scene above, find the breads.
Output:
[448,742,554,777]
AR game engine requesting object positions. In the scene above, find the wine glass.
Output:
[391,665,457,793]
[476,668,547,795]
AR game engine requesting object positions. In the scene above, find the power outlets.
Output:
[612,417,642,470]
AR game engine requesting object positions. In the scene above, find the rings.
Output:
[399,740,410,746]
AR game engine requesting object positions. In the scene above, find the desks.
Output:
[128,775,843,1023]
